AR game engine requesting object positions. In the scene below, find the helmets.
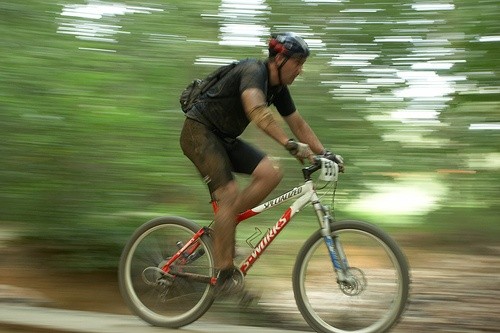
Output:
[267,32,311,85]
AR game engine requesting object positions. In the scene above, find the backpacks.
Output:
[179,57,267,112]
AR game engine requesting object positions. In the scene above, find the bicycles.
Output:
[116,139,409,333]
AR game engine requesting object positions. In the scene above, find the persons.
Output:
[180,32,344,307]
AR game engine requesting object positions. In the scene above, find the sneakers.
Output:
[215,277,262,304]
